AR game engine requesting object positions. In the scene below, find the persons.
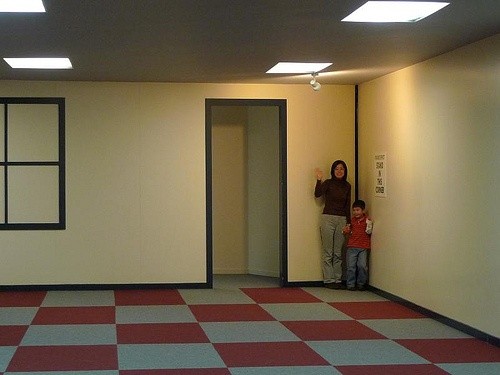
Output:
[315,159,352,289]
[345,199,372,292]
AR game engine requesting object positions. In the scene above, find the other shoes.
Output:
[348,286,356,290]
[359,287,365,291]
[324,282,336,289]
[335,283,346,290]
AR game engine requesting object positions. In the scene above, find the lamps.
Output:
[0,0,449,91]
